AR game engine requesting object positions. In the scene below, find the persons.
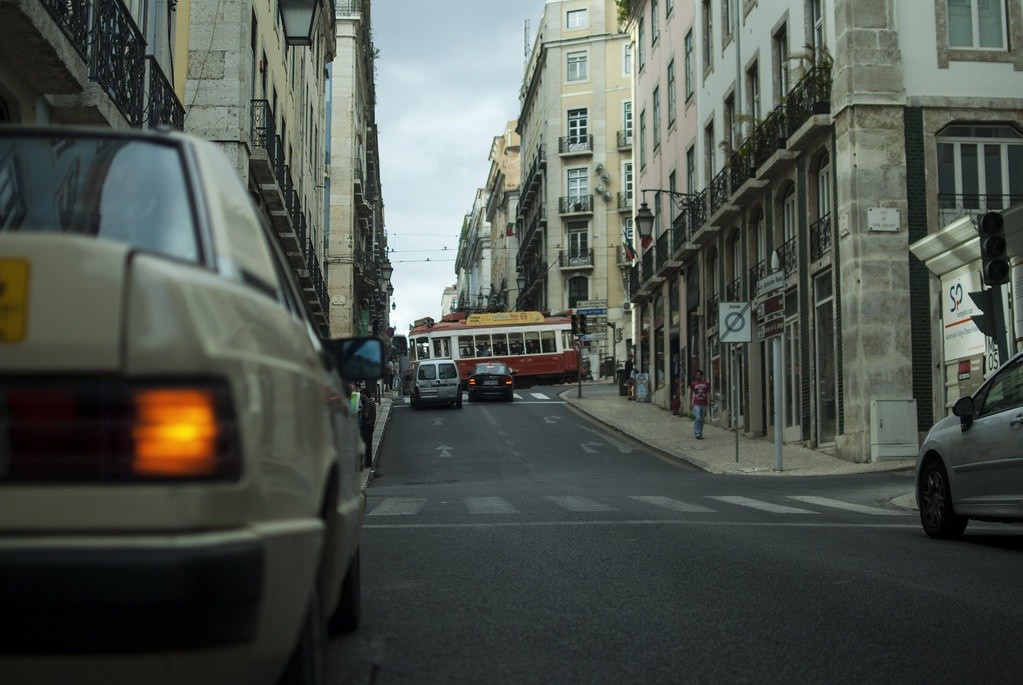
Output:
[359,387,377,467]
[460,339,540,356]
[691,370,711,437]
[383,354,400,393]
[626,353,633,379]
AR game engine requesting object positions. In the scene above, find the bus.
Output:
[406,309,578,390]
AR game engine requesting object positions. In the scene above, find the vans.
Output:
[406,359,462,410]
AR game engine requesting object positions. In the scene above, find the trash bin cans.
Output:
[617,367,633,396]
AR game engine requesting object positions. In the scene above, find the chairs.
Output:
[470,339,552,356]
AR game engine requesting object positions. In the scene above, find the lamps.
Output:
[391,299,397,310]
[636,190,655,240]
[380,253,394,280]
[386,282,394,296]
[477,290,484,305]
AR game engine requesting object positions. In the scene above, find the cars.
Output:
[912,346,1023,543]
[465,363,515,402]
[1,116,380,684]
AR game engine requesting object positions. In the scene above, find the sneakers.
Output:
[696,433,701,438]
